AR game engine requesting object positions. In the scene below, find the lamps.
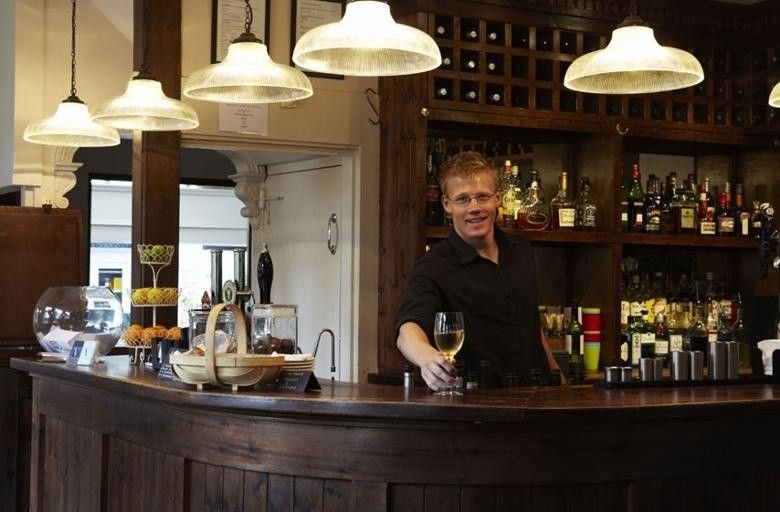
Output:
[561,0,705,95]
[768,81,780,109]
[183,0,314,104]
[23,0,122,148]
[291,0,443,77]
[90,0,200,132]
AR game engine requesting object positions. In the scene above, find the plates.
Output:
[275,354,315,371]
[222,281,237,311]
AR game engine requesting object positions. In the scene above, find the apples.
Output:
[140,245,172,264]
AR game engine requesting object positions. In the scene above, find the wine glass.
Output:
[432,312,466,398]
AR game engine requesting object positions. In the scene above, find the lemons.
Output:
[130,285,180,306]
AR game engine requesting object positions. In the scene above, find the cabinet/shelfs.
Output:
[378,0,780,386]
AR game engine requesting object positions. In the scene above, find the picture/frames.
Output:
[289,0,346,80]
[211,0,270,64]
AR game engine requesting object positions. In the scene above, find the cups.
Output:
[581,306,601,373]
[603,340,741,383]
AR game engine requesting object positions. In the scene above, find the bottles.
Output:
[402,364,414,388]
[565,299,584,377]
[621,265,745,366]
[426,146,598,233]
[435,21,504,108]
[621,164,770,238]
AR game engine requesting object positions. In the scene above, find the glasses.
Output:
[444,191,496,205]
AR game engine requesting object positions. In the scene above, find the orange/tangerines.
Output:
[123,323,182,348]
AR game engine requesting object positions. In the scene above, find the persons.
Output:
[392,150,565,392]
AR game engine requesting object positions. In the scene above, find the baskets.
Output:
[169,303,284,391]
